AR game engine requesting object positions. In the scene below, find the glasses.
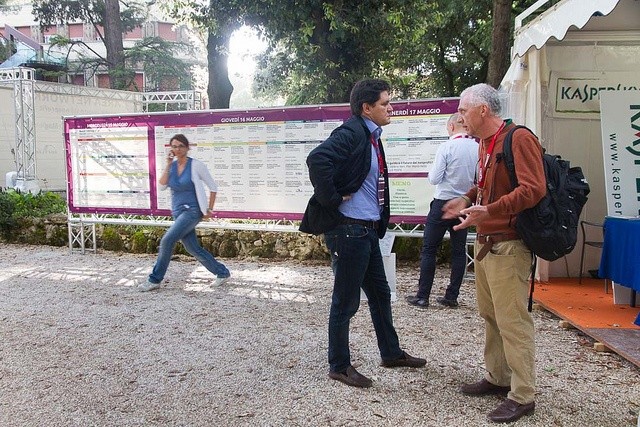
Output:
[171,144,186,148]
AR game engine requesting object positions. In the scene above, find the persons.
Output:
[299,78,427,387]
[441,83,547,424]
[408,113,479,306]
[137,134,231,292]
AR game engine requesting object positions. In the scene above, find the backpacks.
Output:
[503,125,590,262]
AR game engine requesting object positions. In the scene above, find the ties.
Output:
[371,136,386,206]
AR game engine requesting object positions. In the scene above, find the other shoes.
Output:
[210,276,231,287]
[383,351,426,367]
[138,280,160,292]
[329,365,372,387]
[436,297,458,308]
[407,296,429,306]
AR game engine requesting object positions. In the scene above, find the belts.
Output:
[340,219,379,230]
[476,233,521,261]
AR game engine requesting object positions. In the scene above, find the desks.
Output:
[598,215,640,327]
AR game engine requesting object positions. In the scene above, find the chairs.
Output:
[580,219,610,295]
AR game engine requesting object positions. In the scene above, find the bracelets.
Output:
[209,208,212,210]
[461,196,470,206]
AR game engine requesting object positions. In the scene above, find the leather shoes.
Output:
[488,399,535,421]
[461,378,510,395]
